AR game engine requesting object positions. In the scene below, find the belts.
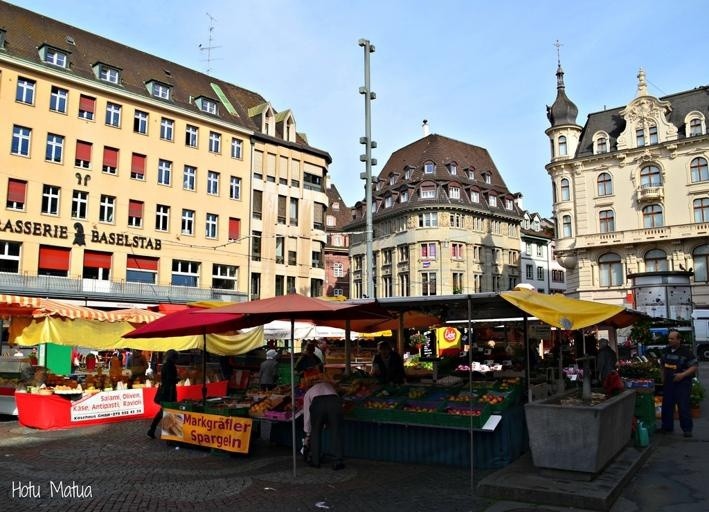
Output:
[313,394,338,401]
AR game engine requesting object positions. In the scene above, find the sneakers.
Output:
[683,430,692,438]
[147,430,156,439]
[332,463,346,471]
[306,460,321,469]
[654,427,674,435]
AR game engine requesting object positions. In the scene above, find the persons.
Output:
[294,343,322,382]
[524,338,541,369]
[302,381,345,468]
[146,352,157,385]
[370,341,407,385]
[257,348,278,392]
[312,340,324,363]
[113,349,123,366]
[598,338,617,382]
[660,331,699,437]
[146,348,184,447]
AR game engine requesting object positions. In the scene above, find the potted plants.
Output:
[690,377,704,418]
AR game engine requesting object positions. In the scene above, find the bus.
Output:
[421,320,556,364]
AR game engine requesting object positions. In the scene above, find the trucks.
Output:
[691,307,709,360]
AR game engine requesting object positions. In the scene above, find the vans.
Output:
[624,327,692,359]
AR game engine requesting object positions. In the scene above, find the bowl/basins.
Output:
[39,390,54,395]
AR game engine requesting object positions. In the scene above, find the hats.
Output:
[265,349,278,360]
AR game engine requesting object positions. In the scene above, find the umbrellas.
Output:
[191,292,360,482]
[120,309,271,405]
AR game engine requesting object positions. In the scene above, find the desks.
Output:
[14,380,229,431]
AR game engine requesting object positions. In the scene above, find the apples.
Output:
[285,397,304,414]
[362,391,503,417]
